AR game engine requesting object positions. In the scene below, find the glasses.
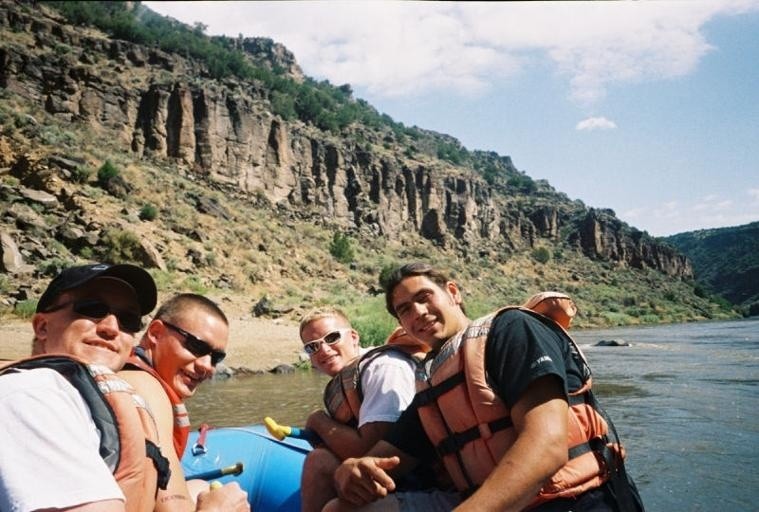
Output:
[158,318,227,365]
[302,328,353,355]
[39,294,144,335]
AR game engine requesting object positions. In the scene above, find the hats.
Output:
[34,262,157,316]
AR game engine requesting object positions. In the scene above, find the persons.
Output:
[320,261,635,510]
[0,260,255,512]
[293,303,423,512]
[119,290,231,506]
